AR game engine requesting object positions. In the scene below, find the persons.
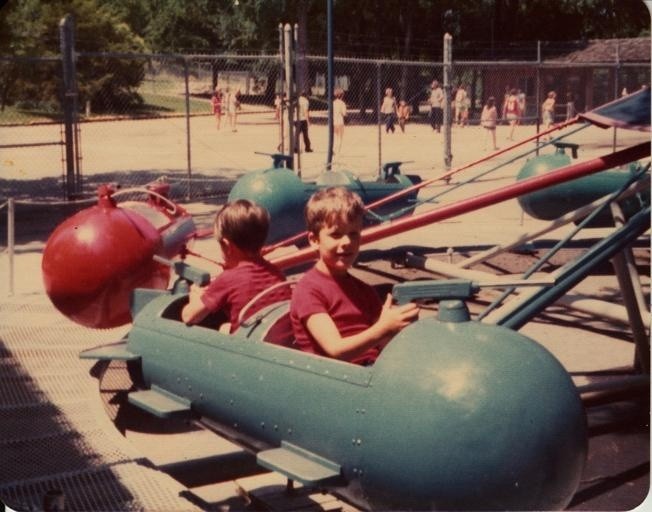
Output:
[288,188,420,367]
[397,99,410,132]
[565,85,576,122]
[181,199,292,336]
[427,80,444,133]
[211,84,243,132]
[450,83,526,152]
[276,90,313,154]
[381,88,398,134]
[330,87,348,156]
[541,90,557,142]
